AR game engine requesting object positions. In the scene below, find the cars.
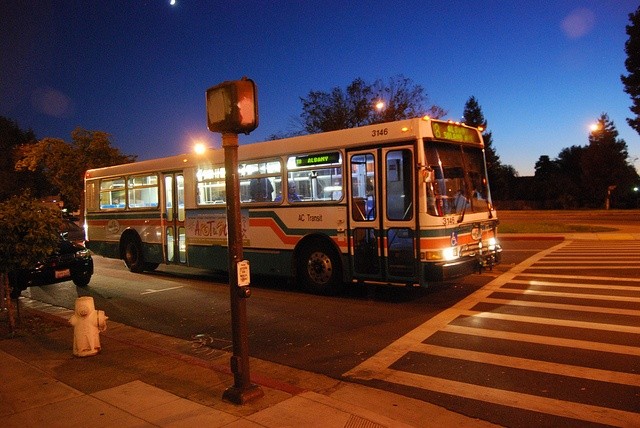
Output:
[50,220,85,245]
[5,234,93,298]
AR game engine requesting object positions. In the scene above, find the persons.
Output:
[456,186,484,214]
[274,178,302,202]
[248,171,274,201]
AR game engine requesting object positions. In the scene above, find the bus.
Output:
[84,116,502,293]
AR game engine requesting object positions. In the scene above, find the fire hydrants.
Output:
[67,296,109,358]
